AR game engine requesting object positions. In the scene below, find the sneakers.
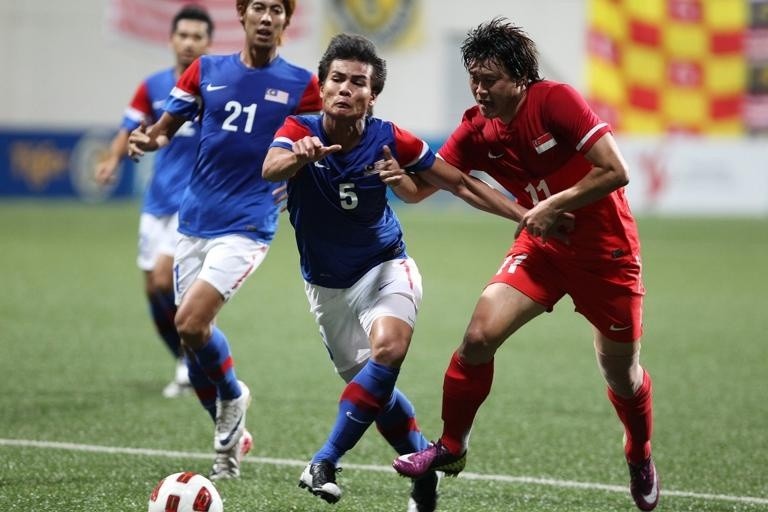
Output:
[407,469,443,511]
[209,379,252,481]
[298,458,342,504]
[393,438,468,478]
[628,455,659,512]
[162,358,192,397]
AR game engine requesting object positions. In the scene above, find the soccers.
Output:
[147,472,224,512]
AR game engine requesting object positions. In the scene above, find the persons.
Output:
[88,3,218,403]
[117,0,321,483]
[258,32,578,512]
[370,14,664,512]
